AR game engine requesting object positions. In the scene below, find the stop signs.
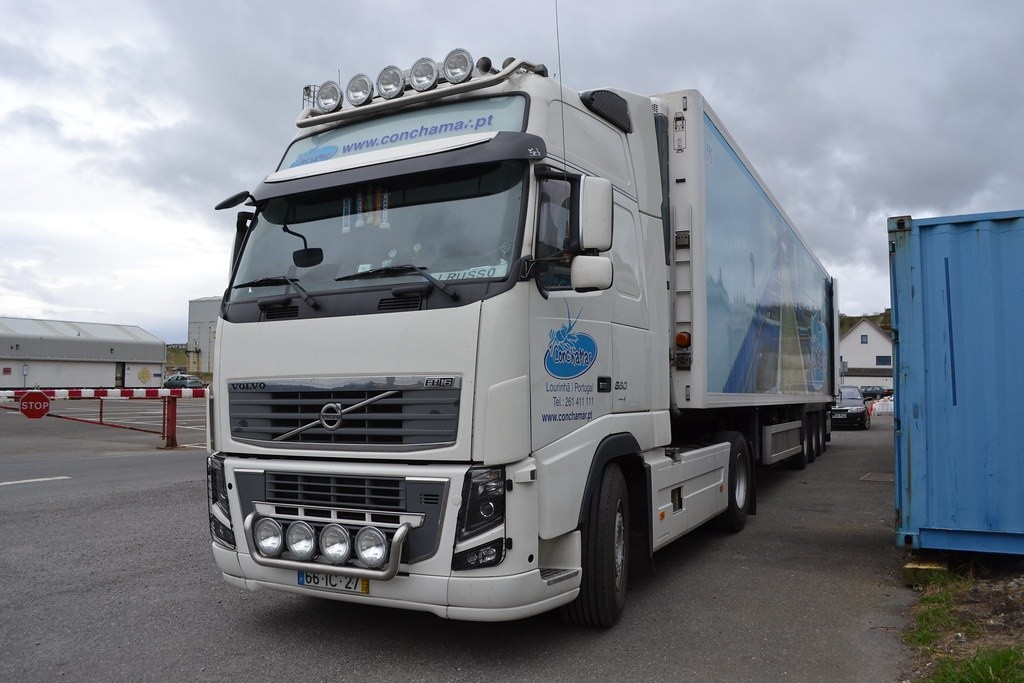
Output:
[19,391,50,420]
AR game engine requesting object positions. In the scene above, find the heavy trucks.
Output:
[204,47,843,635]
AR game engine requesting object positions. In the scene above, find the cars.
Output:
[164,374,203,388]
[860,385,893,400]
[831,385,871,429]
